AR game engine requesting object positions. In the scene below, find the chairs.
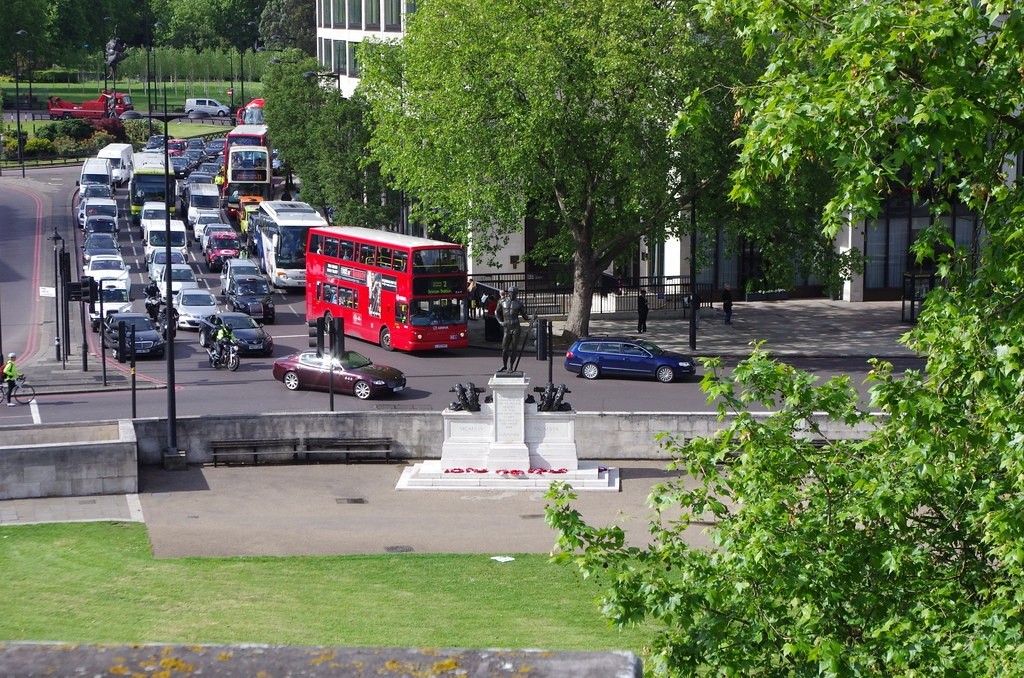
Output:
[314,245,352,259]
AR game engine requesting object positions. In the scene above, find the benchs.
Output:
[209,438,300,464]
[302,438,392,464]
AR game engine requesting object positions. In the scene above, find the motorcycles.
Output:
[144,291,177,341]
[205,329,240,371]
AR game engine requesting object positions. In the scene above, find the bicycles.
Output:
[0,373,35,405]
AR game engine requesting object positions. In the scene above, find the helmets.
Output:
[226,323,233,331]
[150,280,157,284]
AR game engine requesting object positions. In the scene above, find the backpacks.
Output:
[0,362,13,380]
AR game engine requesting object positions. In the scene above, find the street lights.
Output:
[304,71,341,99]
[47,227,64,361]
[102,16,163,137]
[225,21,257,108]
[14,30,35,162]
[119,109,211,453]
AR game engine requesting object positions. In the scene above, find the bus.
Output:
[220,99,273,201]
[305,224,468,350]
[253,200,330,288]
[127,151,176,225]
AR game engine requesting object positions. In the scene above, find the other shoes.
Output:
[725,319,733,325]
[7,403,16,406]
[211,363,215,367]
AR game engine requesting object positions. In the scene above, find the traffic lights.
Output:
[308,318,318,347]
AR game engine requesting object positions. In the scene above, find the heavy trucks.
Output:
[48,91,134,120]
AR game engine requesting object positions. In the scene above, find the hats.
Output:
[8,353,16,358]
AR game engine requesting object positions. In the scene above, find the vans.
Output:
[75,142,135,199]
[185,99,230,116]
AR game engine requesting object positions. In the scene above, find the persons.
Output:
[212,324,238,368]
[722,284,733,325]
[162,303,179,317]
[281,190,292,201]
[144,280,160,313]
[0,353,22,407]
[294,189,301,201]
[495,287,531,373]
[214,172,224,190]
[637,290,649,334]
[317,243,321,254]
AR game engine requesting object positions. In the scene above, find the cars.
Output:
[564,336,696,383]
[77,132,277,355]
[272,350,407,400]
